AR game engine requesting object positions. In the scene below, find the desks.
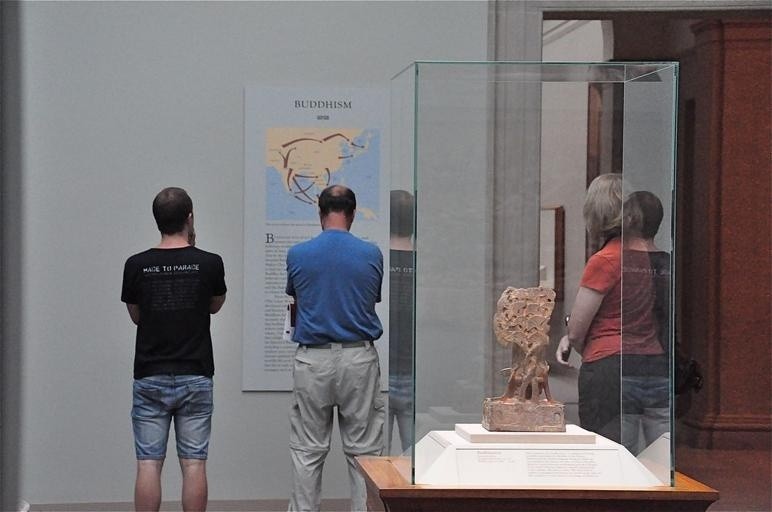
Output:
[350,453,720,511]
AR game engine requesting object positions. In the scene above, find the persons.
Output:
[556,174,668,457]
[387,189,416,456]
[623,190,671,357]
[120,187,227,512]
[285,186,386,512]
